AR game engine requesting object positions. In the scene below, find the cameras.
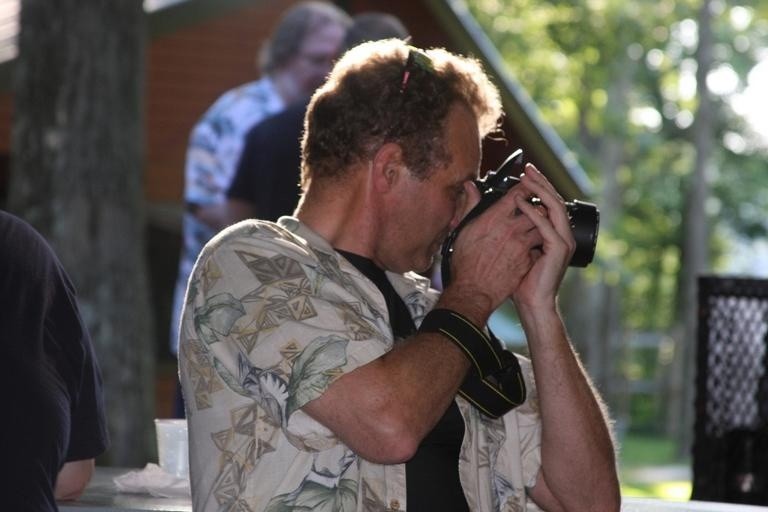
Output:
[459,177,598,268]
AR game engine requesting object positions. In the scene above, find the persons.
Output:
[178,36,620,512]
[169,0,355,418]
[0,210,110,512]
[223,12,411,224]
